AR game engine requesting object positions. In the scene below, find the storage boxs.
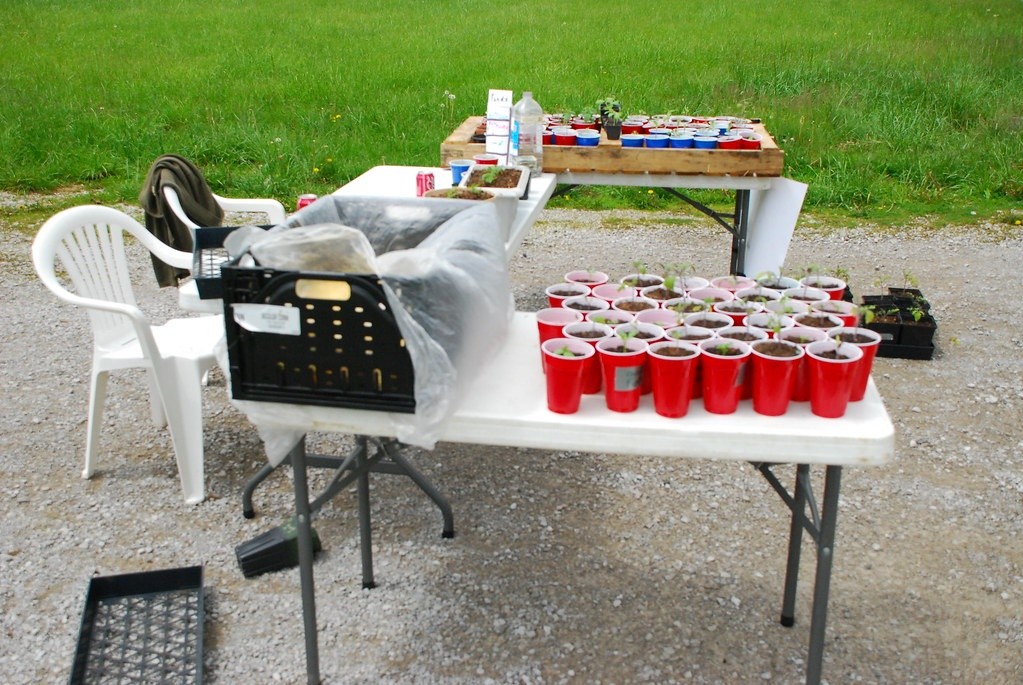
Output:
[439,116,784,177]
[221,195,497,415]
[542,115,763,150]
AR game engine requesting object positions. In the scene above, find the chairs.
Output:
[136,158,284,385]
[32,204,225,507]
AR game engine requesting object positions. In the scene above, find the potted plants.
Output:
[535,268,938,418]
[461,165,530,196]
[423,189,495,202]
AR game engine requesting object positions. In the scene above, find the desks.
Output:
[179,167,556,539]
[557,179,773,276]
[227,308,899,685]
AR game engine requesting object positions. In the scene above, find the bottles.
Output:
[508,92,543,177]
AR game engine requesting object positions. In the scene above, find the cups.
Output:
[473,155,499,165]
[535,308,584,375]
[450,160,477,184]
[592,283,638,308]
